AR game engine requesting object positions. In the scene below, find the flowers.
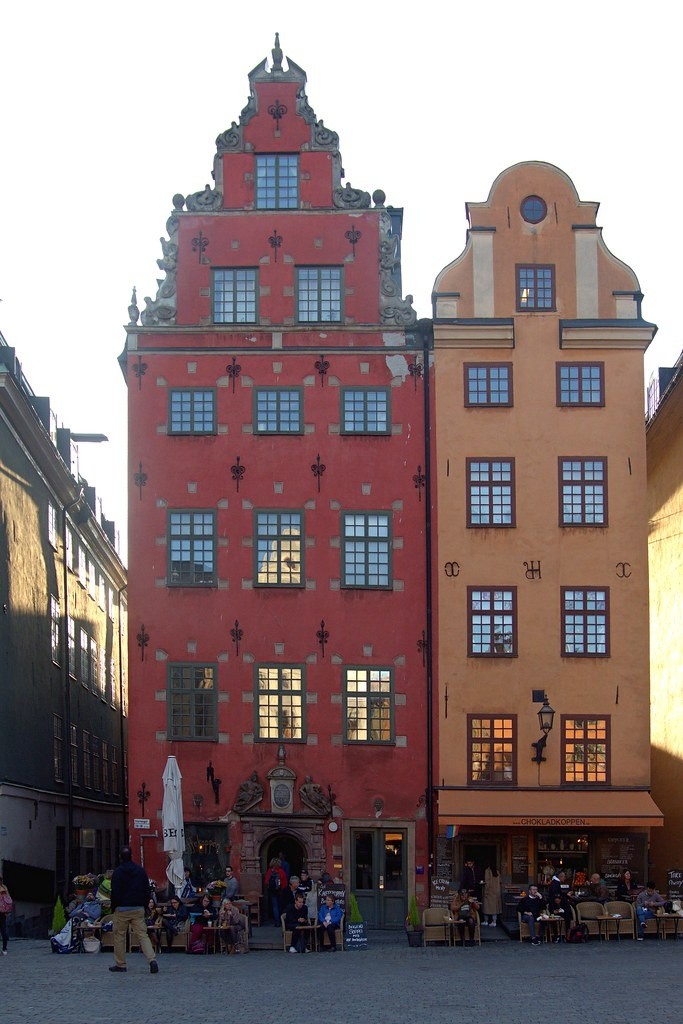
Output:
[207,880,227,894]
[72,875,90,890]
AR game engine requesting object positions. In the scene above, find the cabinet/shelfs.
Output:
[235,894,265,928]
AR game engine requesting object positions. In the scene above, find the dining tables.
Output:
[541,916,564,943]
[146,927,164,955]
[203,925,232,956]
[76,925,103,954]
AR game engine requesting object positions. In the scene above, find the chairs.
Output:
[518,901,683,942]
[282,912,345,952]
[100,909,249,953]
[423,908,481,947]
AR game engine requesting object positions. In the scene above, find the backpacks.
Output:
[268,868,281,890]
[566,923,590,943]
[0,894,13,912]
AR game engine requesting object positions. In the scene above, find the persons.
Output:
[449,889,480,947]
[50,846,343,973]
[460,859,484,890]
[482,859,503,927]
[517,870,667,946]
[0,874,14,955]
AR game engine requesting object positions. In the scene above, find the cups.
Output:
[208,921,212,927]
[310,918,316,926]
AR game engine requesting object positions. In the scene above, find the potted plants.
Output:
[50,896,66,952]
[407,896,422,946]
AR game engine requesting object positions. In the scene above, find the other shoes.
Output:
[230,945,235,953]
[109,966,127,972]
[150,961,158,973]
[531,939,541,945]
[462,939,476,947]
[489,922,496,927]
[288,947,297,953]
[1,950,7,955]
[641,922,648,928]
[304,949,311,953]
[328,947,337,951]
[637,937,644,940]
[481,921,488,925]
[554,935,560,943]
[318,946,324,952]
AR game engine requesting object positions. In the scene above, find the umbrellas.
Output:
[161,755,185,900]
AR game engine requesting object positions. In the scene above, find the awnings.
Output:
[436,789,664,827]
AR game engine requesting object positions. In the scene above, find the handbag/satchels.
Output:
[83,936,100,953]
[234,943,249,954]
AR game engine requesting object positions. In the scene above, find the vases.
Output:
[76,890,88,896]
[212,896,221,906]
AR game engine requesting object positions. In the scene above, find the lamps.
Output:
[531,694,556,765]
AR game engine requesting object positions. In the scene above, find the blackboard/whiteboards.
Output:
[510,833,528,884]
[317,883,346,918]
[664,869,683,900]
[346,921,367,949]
[593,832,648,888]
[435,834,454,882]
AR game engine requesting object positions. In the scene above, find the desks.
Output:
[296,926,320,952]
[654,913,682,945]
[596,916,622,944]
[441,920,466,948]
